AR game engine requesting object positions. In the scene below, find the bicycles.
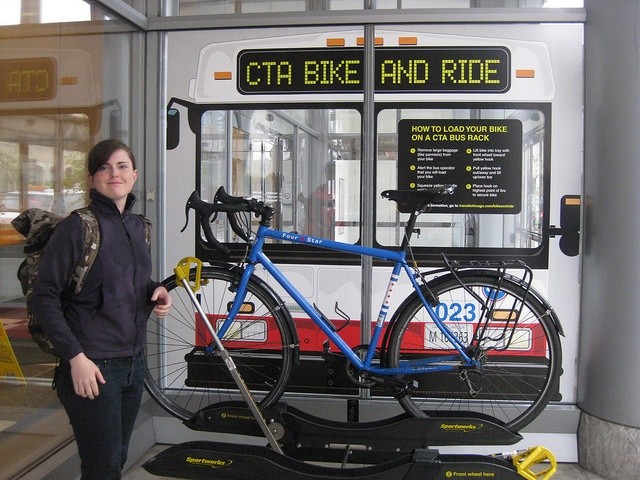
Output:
[139,187,565,435]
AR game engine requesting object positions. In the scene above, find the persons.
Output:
[31,139,173,479]
[304,159,336,242]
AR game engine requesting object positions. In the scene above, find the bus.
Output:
[162,28,584,394]
[0,45,120,348]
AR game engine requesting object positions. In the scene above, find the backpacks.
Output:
[11,208,152,359]
